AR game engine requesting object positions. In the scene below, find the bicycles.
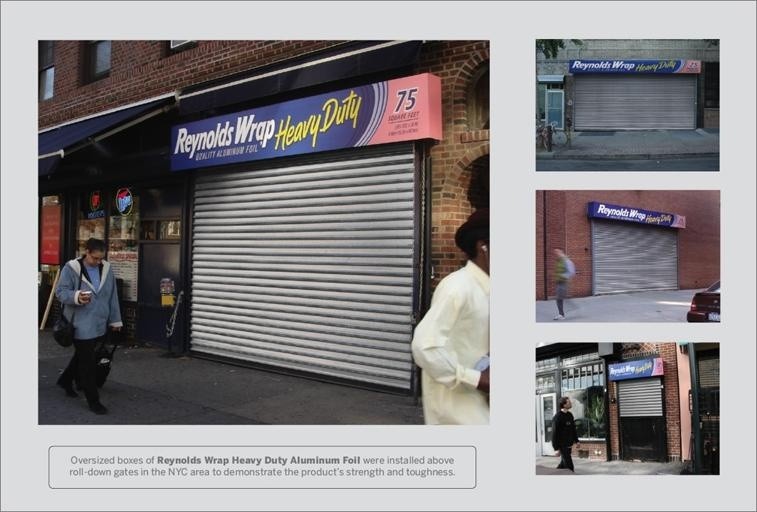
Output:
[550,120,568,148]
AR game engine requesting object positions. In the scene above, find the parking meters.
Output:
[566,115,572,148]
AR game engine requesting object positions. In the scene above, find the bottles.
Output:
[475,351,489,371]
[109,242,137,262]
[75,241,89,257]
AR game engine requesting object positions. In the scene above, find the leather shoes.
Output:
[57,377,108,415]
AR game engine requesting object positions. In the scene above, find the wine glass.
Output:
[160,219,182,240]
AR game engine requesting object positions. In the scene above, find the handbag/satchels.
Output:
[52,316,74,347]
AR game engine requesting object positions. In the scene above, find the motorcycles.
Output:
[537,118,554,152]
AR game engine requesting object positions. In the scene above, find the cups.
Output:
[76,215,138,240]
[80,291,92,303]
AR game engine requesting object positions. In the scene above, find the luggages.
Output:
[75,326,120,390]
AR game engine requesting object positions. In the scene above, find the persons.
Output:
[409,209,490,427]
[550,397,580,472]
[55,236,123,415]
[553,246,576,322]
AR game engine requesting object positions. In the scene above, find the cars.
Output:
[687,280,720,323]
[574,418,604,438]
